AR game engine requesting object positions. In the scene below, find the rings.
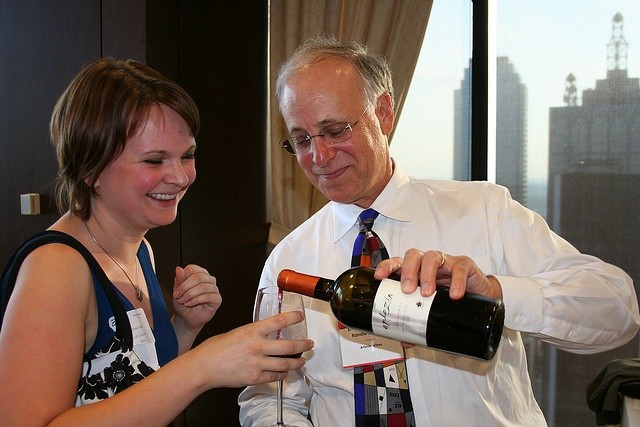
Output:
[435,250,447,270]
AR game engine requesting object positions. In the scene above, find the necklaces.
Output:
[68,199,144,303]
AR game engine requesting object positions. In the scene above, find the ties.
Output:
[351,209,415,427]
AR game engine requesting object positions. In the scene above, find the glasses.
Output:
[278,101,375,155]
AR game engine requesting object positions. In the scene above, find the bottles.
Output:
[276,270,505,360]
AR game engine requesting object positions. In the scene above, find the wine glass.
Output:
[254,286,309,427]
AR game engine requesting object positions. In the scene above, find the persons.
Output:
[0,56,314,426]
[238,35,572,426]
